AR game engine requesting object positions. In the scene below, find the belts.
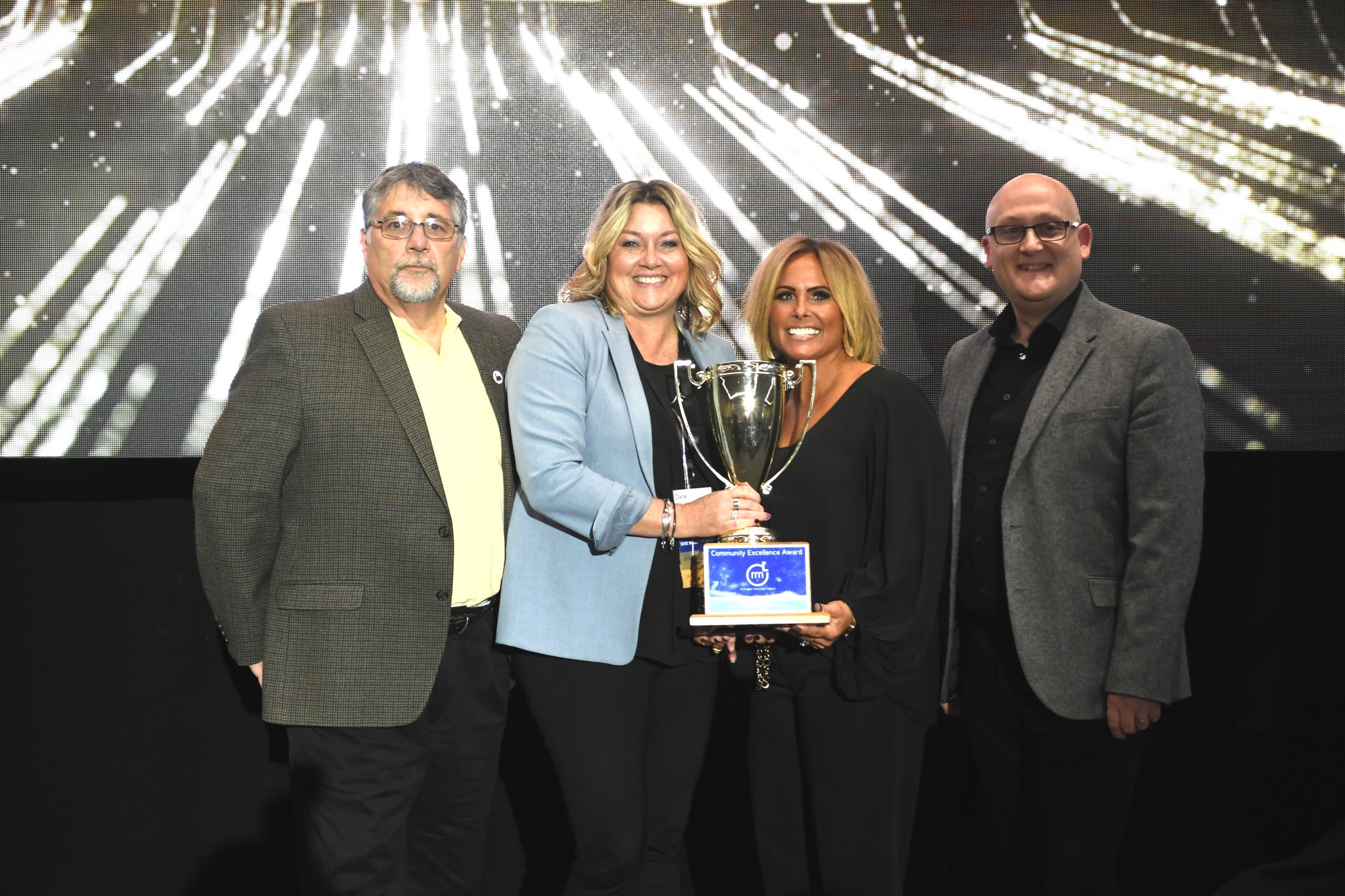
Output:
[446,598,497,636]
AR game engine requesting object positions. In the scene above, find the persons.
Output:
[502,178,778,895]
[190,161,528,896]
[734,232,948,896]
[934,172,1207,896]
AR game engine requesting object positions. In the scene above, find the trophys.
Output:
[666,349,826,626]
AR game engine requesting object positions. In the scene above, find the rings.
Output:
[798,639,808,646]
[732,510,738,519]
[713,647,722,654]
[1136,718,1149,724]
[733,498,739,510]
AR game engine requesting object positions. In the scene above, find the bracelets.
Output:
[659,496,677,551]
[843,612,857,639]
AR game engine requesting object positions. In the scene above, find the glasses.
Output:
[987,221,1081,246]
[365,216,459,239]
